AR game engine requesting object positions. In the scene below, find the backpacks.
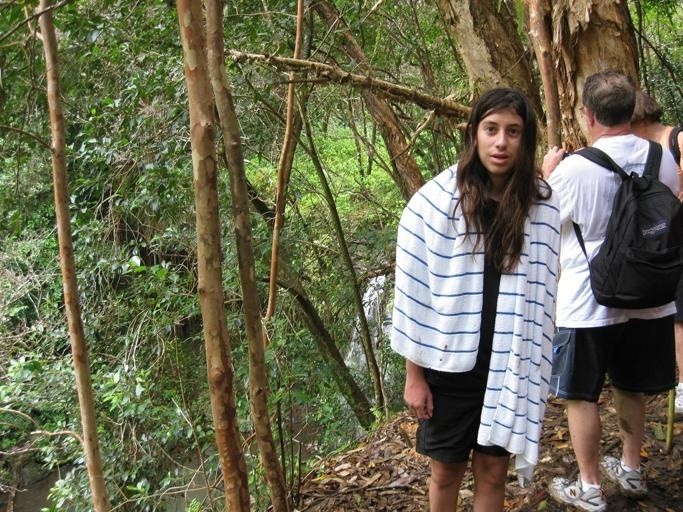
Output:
[570,139,682,309]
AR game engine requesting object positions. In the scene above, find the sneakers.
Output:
[602,456,647,494]
[674,384,683,413]
[549,477,607,511]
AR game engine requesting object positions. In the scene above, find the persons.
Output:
[539,71,680,512]
[630,90,683,424]
[390,89,560,512]
